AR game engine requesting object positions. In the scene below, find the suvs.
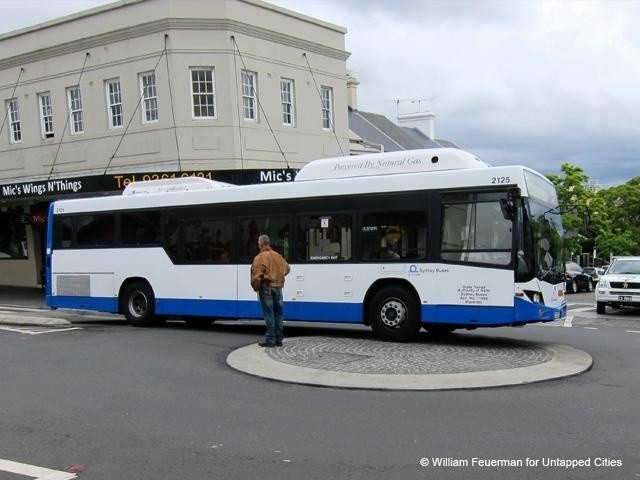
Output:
[582,267,607,289]
[594,255,639,315]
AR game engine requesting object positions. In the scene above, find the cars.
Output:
[563,261,593,293]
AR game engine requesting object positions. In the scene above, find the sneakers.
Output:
[259,341,275,347]
[276,339,282,346]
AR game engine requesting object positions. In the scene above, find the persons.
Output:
[131,219,290,264]
[250,234,290,347]
[378,229,404,259]
[539,230,557,274]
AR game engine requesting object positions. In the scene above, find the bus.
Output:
[44,149,592,345]
